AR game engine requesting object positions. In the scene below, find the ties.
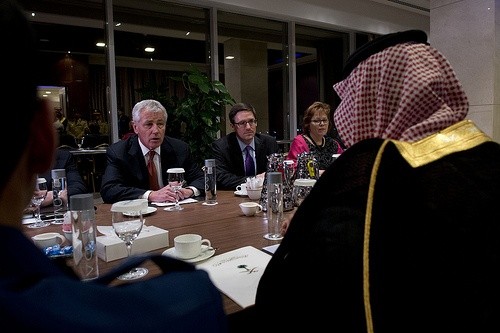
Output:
[146,150,160,192]
[244,145,256,181]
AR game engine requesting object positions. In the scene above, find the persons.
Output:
[254,29,500,333]
[50,103,131,176]
[207,102,281,191]
[0,69,228,333]
[285,102,345,174]
[34,146,88,202]
[99,99,207,204]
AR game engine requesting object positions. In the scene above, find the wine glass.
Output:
[112,209,149,280]
[26,178,50,228]
[166,168,185,210]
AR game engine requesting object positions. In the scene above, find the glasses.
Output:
[310,120,328,124]
[233,120,257,127]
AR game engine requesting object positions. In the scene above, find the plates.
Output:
[122,207,157,216]
[160,245,215,263]
[234,190,248,197]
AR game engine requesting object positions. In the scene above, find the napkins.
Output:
[150,198,198,207]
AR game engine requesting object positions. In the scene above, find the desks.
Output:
[65,147,108,194]
[22,189,303,317]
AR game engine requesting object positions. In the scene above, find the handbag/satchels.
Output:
[89,252,228,333]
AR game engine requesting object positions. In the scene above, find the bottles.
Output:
[51,169,69,224]
[205,159,216,204]
[267,172,283,238]
[70,194,99,281]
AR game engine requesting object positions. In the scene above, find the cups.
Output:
[129,199,148,212]
[292,179,317,207]
[239,202,263,216]
[31,233,65,249]
[174,234,211,259]
[236,183,247,193]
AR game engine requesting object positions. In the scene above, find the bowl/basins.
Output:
[246,189,262,200]
